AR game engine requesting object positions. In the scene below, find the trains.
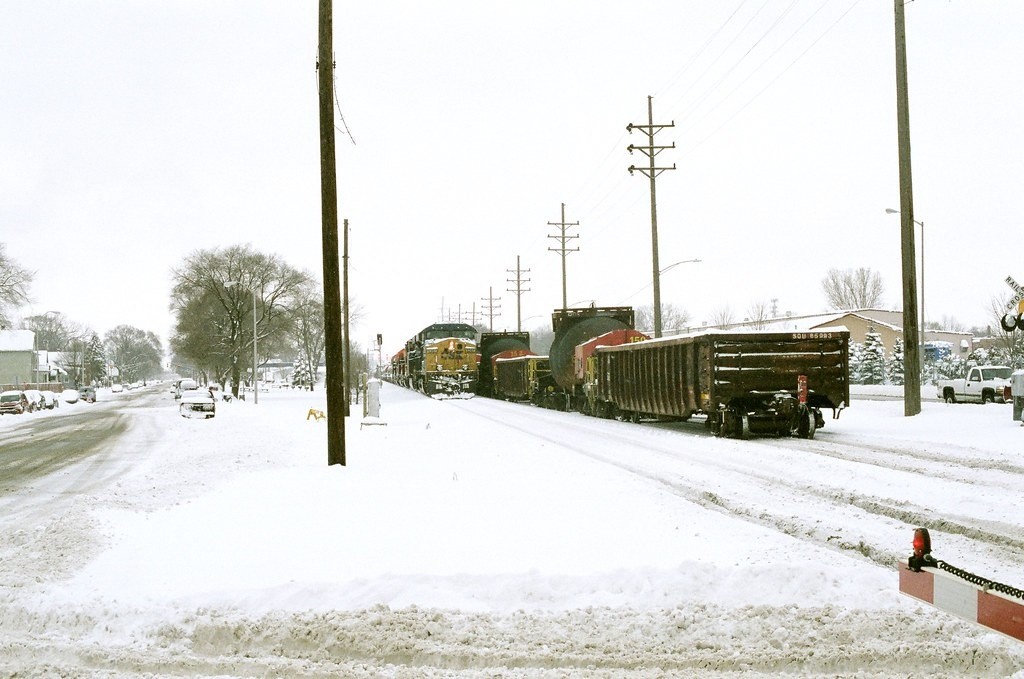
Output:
[477,305,850,438]
[373,323,480,400]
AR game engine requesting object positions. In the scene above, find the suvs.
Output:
[0,391,31,414]
[173,378,200,400]
[80,387,96,402]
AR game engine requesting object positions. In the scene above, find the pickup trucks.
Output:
[936,364,1013,405]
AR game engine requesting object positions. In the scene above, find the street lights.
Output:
[36,310,61,390]
[121,352,131,384]
[653,258,699,339]
[82,332,98,386]
[885,208,926,380]
[224,281,258,405]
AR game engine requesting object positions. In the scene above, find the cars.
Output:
[62,390,79,403]
[42,391,59,409]
[180,390,216,418]
[127,380,162,390]
[111,384,123,393]
[171,383,176,393]
[24,390,45,411]
